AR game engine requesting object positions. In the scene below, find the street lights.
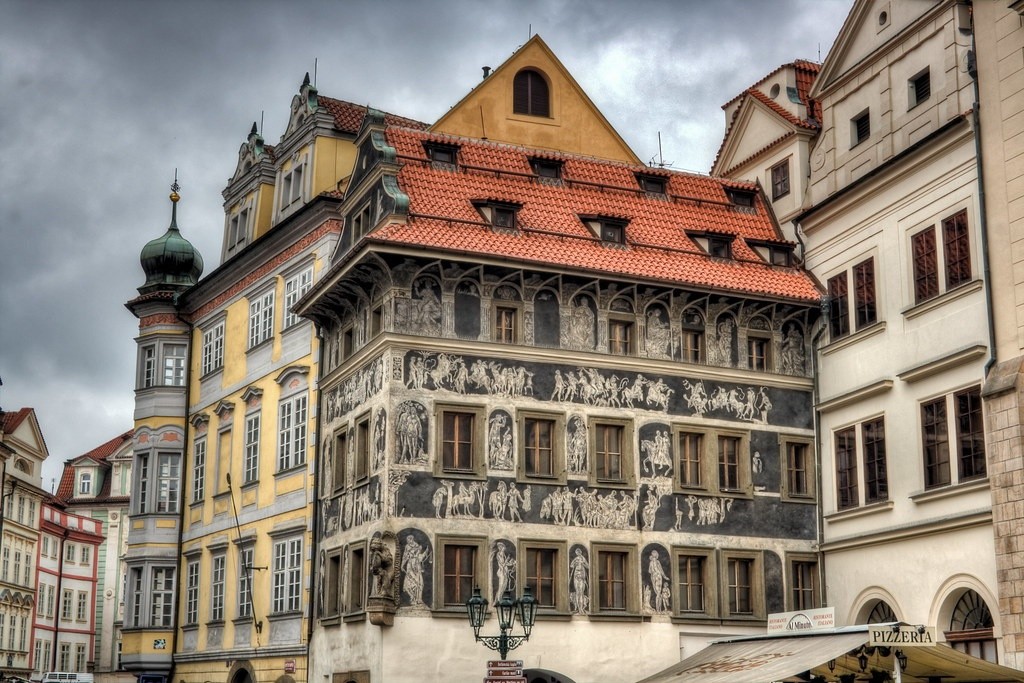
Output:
[465,582,540,683]
[53,529,71,670]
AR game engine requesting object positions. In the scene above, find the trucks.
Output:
[138,673,168,683]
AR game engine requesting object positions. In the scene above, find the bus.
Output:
[42,672,94,683]
